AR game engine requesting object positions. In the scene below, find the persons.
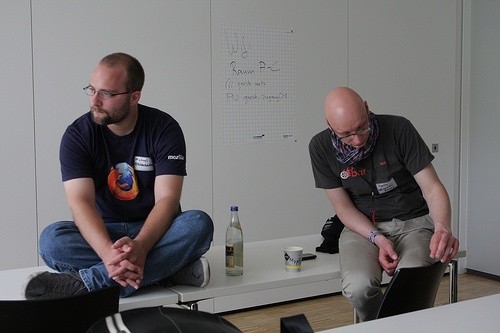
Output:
[24,53,215,300]
[309,87,460,323]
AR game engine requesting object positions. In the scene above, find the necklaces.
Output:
[353,155,377,225]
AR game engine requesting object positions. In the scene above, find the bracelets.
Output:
[368,231,384,244]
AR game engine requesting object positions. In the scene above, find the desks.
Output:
[0,233,500,333]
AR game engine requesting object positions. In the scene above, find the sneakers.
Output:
[162,257,210,287]
[25,271,89,300]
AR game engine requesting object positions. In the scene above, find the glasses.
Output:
[82,86,136,100]
[328,122,371,143]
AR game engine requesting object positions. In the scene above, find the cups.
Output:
[283,247,303,272]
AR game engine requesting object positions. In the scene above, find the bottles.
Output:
[225,206,244,277]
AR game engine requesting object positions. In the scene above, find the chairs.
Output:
[375,260,449,319]
[0,285,120,333]
[86,307,243,333]
